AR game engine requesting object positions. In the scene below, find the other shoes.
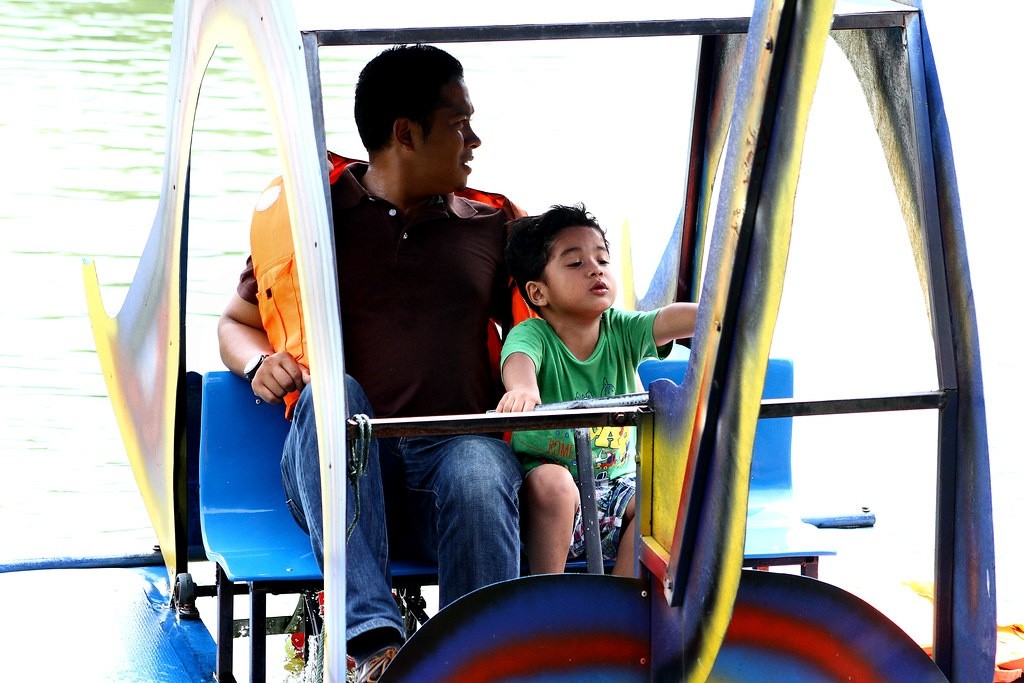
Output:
[347,643,400,683]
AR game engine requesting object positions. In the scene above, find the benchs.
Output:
[199,359,839,683]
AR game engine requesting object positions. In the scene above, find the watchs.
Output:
[243,354,269,379]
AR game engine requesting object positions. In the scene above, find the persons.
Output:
[217,45,531,683]
[497,205,701,577]
